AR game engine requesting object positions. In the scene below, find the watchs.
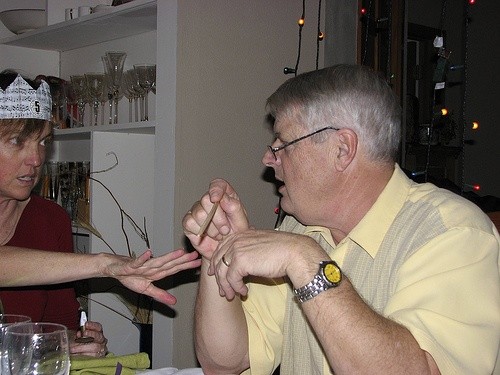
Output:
[294,261,344,303]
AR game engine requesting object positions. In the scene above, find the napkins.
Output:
[40,351,150,375]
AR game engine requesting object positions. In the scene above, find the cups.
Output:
[65,6,92,22]
[0,314,70,375]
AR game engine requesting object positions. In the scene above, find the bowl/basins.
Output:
[0,8,47,35]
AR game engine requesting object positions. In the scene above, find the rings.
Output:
[222,255,230,267]
[99,344,105,354]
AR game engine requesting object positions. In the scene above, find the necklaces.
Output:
[0,206,19,245]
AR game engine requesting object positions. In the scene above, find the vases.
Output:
[133,318,152,369]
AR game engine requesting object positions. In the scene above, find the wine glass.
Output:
[40,161,91,226]
[63,52,157,128]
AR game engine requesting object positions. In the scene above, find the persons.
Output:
[184,63,500,375]
[0,71,203,357]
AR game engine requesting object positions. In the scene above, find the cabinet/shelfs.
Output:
[0,0,158,356]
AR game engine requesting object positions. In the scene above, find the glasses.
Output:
[267,126,339,161]
